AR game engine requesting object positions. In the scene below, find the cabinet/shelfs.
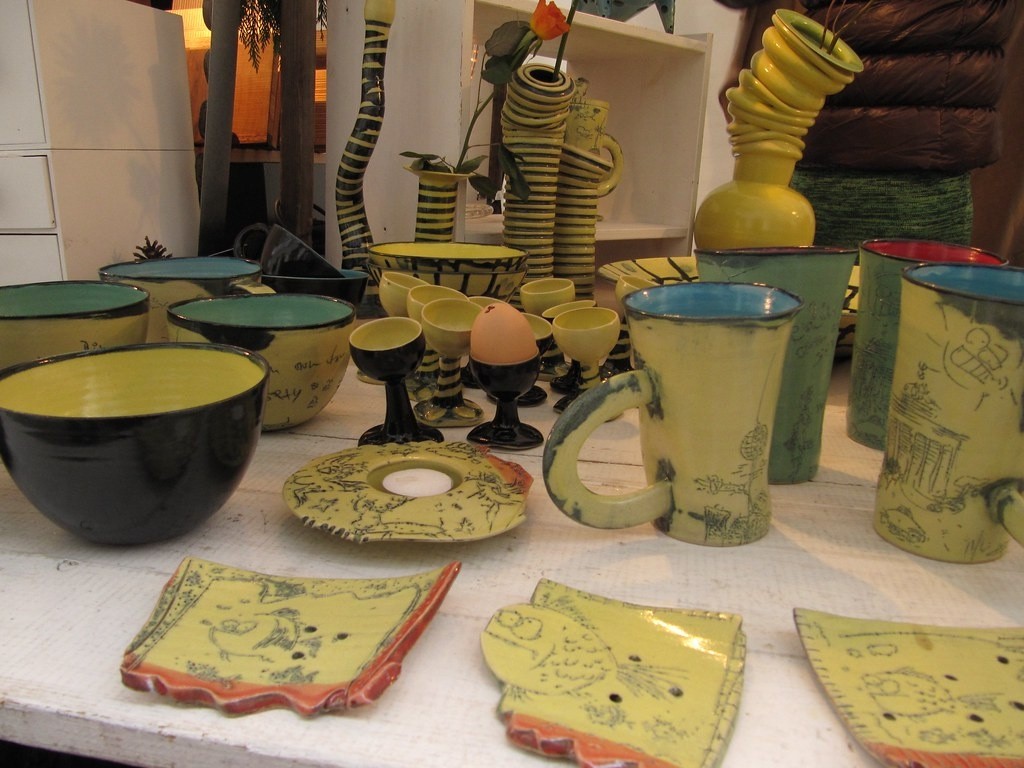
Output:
[0,1,200,285]
[362,2,713,317]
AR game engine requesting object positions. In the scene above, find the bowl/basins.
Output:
[163,292,358,433]
[262,269,368,306]
[99,258,276,350]
[0,342,269,546]
[367,240,529,299]
[0,279,150,375]
[599,258,859,360]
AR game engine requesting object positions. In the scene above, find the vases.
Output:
[400,168,476,241]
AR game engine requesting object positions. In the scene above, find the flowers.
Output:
[401,1,567,197]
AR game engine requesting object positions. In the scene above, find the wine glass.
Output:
[348,272,621,450]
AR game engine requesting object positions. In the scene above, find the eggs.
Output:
[471,302,536,367]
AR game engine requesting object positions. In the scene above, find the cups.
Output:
[233,224,346,277]
[541,280,806,549]
[871,262,1024,564]
[694,245,860,484]
[848,240,1011,451]
